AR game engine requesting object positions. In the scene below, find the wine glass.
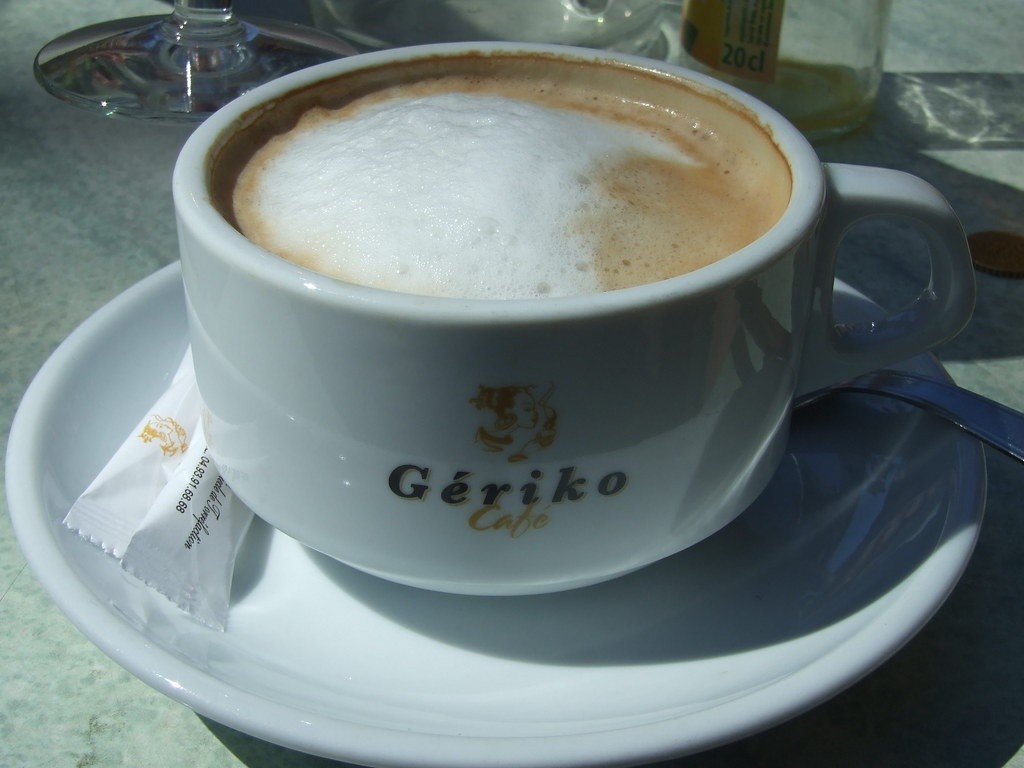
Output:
[34,0,361,124]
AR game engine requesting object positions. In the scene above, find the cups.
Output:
[172,44,973,593]
[679,1,891,139]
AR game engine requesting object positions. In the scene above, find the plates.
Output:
[9,259,989,768]
